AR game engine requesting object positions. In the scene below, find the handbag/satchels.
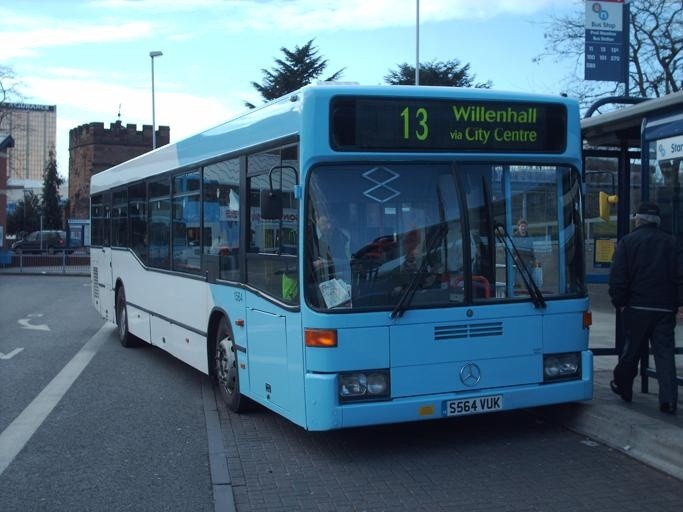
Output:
[531,264,544,288]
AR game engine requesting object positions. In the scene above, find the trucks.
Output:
[136,171,221,244]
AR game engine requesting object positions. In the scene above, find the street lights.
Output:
[150,51,163,150]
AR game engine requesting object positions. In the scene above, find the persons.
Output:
[512,220,540,290]
[608,203,683,412]
[308,206,348,268]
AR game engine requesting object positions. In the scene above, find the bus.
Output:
[90,84,594,432]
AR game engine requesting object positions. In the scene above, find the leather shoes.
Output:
[659,400,678,414]
[610,380,632,403]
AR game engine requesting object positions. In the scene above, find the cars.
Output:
[11,230,67,254]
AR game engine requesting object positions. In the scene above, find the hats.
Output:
[633,202,660,217]
